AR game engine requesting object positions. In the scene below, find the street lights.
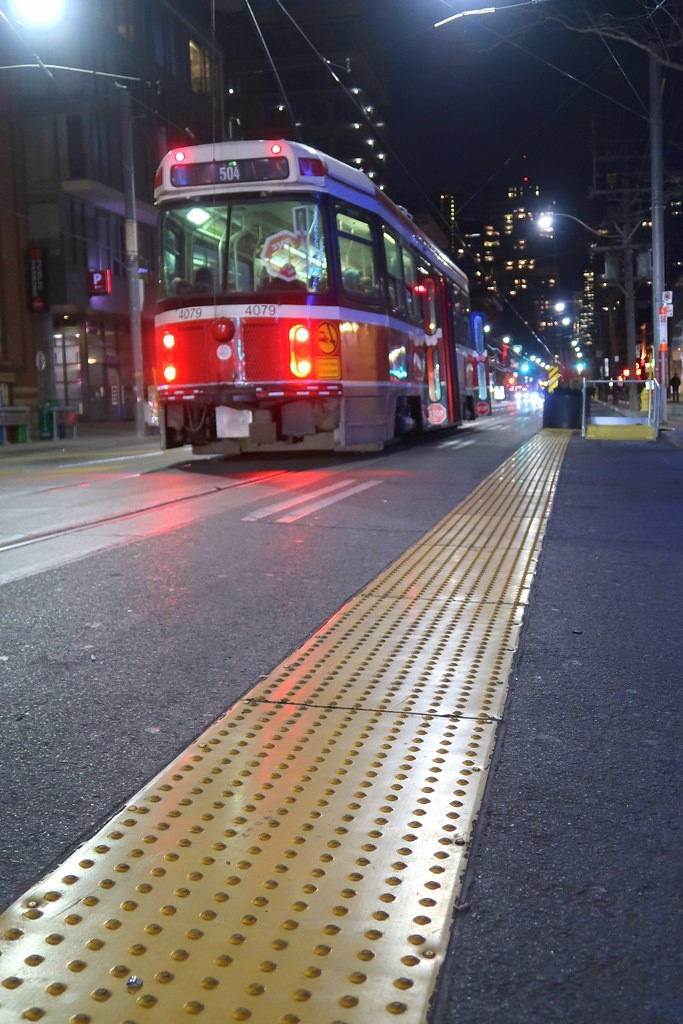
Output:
[537,213,644,418]
[554,300,618,406]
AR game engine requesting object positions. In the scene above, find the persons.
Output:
[272,264,306,291]
[343,269,371,294]
[172,268,216,294]
[670,373,681,402]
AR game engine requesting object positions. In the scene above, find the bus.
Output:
[155,139,477,459]
